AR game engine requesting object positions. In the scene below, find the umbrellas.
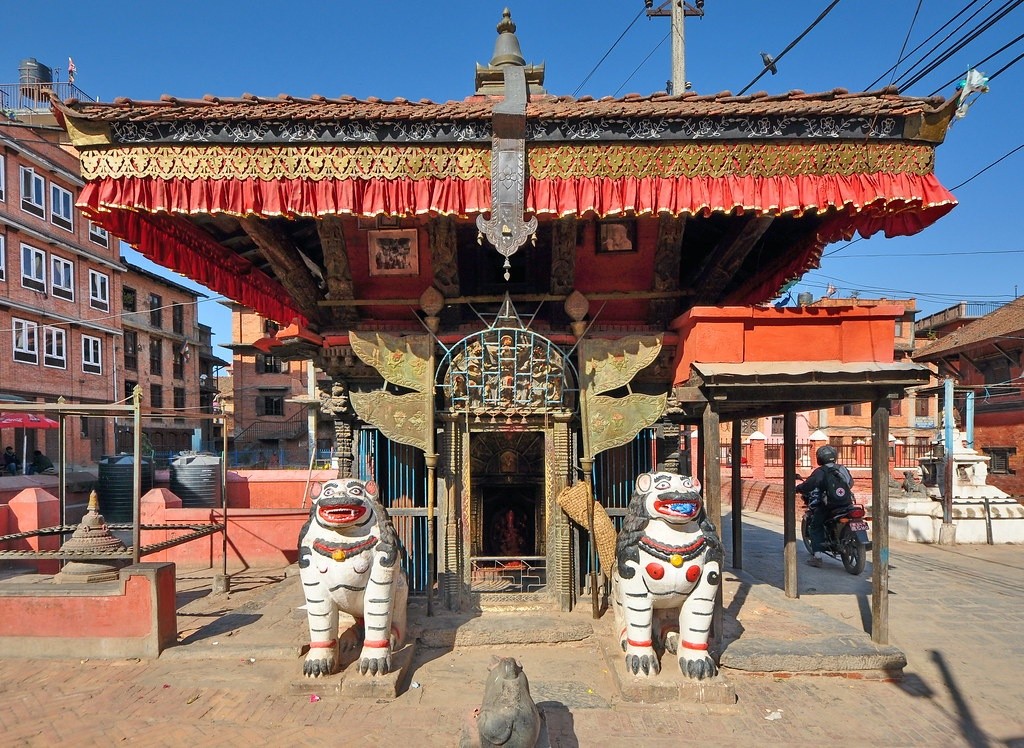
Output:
[0,413,59,475]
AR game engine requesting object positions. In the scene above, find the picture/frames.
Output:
[594,216,639,254]
[366,227,420,279]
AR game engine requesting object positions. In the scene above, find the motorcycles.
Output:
[794,473,872,576]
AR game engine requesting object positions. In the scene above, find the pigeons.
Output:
[759,51,777,75]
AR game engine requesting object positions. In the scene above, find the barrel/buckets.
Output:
[170,455,221,508]
[99,453,156,523]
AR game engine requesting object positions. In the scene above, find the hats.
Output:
[6,446,13,451]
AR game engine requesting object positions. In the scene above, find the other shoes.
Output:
[806,555,823,568]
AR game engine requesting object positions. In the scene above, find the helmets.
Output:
[816,445,838,466]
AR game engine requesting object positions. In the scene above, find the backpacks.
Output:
[820,465,852,512]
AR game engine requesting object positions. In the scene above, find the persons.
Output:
[4,446,23,476]
[28,451,55,475]
[255,451,279,470]
[796,446,854,566]
